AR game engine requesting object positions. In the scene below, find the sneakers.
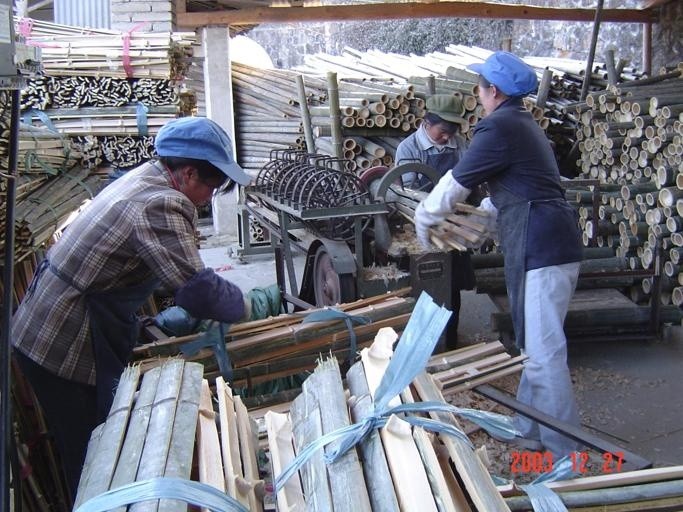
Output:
[488,424,542,453]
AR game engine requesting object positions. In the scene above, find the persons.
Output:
[9,114,254,500]
[395,93,481,218]
[414,50,586,459]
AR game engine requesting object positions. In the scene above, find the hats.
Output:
[423,93,468,126]
[464,50,537,98]
[152,117,251,189]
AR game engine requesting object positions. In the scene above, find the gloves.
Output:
[413,201,436,250]
